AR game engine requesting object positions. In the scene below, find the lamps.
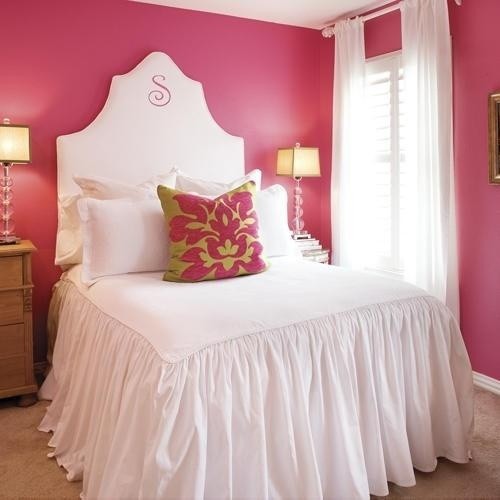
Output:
[275,145,323,249]
[0,116,31,247]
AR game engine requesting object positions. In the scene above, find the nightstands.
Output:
[0,241,41,407]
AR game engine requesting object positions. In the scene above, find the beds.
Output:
[46,48,471,499]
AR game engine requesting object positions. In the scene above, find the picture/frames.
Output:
[489,93,500,185]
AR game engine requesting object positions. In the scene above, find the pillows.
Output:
[55,167,301,292]
